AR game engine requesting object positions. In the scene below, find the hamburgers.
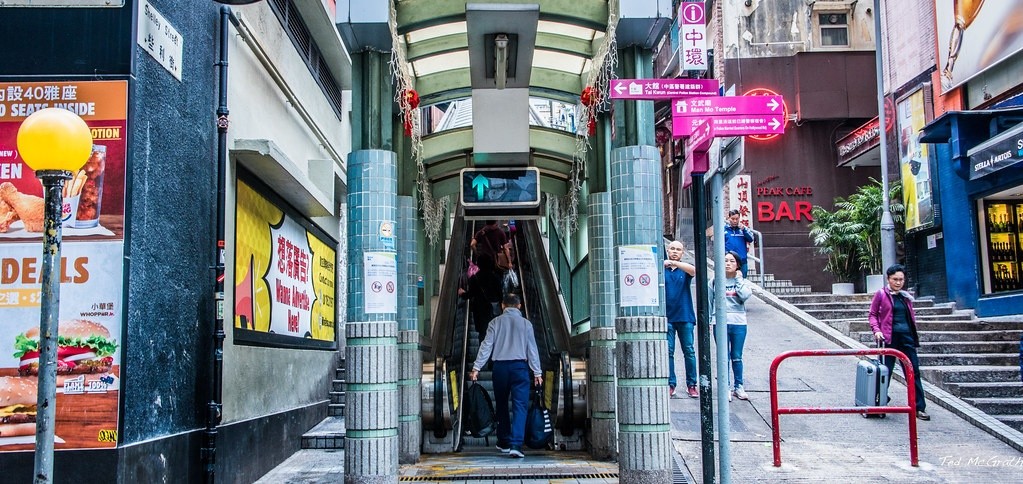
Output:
[0,319,121,437]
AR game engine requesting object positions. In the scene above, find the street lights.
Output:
[17,107,93,484]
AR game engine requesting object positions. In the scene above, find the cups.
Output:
[75,144,107,228]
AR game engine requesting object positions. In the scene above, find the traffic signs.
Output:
[609,78,785,189]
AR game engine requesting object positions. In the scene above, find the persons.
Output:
[868,264,931,421]
[724,210,754,279]
[469,293,544,458]
[663,240,700,398]
[708,250,753,403]
[470,220,516,303]
[458,265,496,371]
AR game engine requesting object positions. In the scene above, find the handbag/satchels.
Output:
[504,267,519,288]
[524,384,553,449]
[495,252,509,271]
[510,225,517,232]
[488,301,501,319]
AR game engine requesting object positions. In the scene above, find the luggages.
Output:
[853,339,889,419]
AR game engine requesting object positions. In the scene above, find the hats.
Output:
[485,220,497,225]
[502,221,509,227]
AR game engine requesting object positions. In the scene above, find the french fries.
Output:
[60,168,89,198]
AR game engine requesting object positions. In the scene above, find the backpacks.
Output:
[464,381,499,447]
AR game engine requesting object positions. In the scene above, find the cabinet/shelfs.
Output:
[984,198,1023,294]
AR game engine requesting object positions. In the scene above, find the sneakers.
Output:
[509,445,524,458]
[495,441,511,453]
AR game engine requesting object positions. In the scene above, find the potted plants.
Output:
[807,176,903,296]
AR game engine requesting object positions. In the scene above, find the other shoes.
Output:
[733,386,748,399]
[915,410,930,420]
[728,389,732,402]
[670,386,676,398]
[686,385,699,397]
